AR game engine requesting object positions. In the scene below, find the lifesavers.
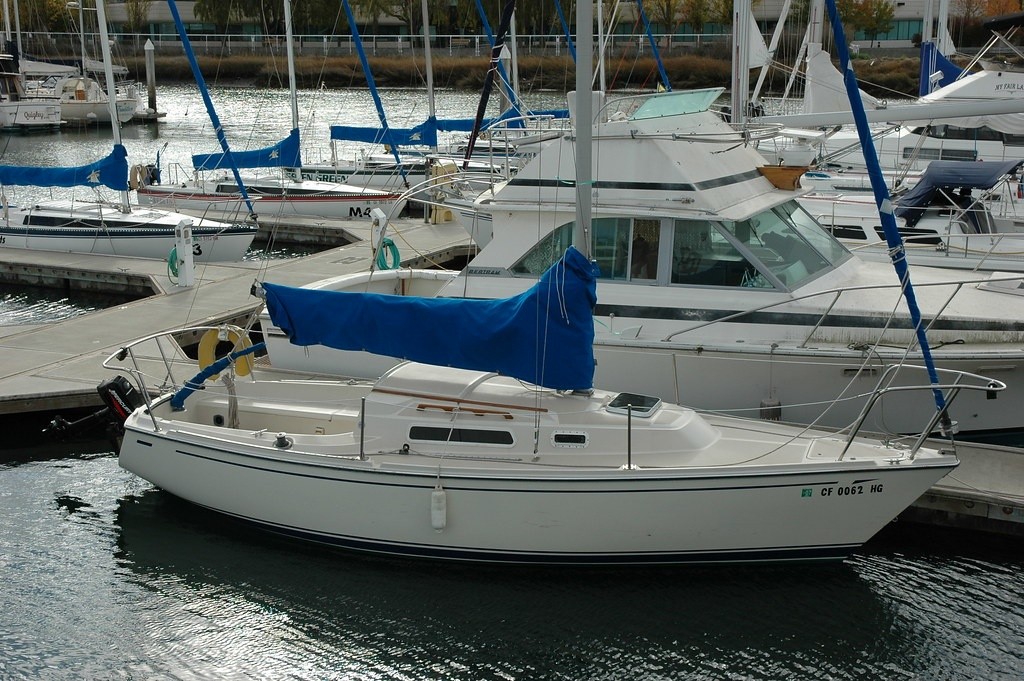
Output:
[376,237,400,270]
[198,325,255,380]
[131,164,148,189]
[169,248,179,276]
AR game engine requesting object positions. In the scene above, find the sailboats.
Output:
[0,0,1023,567]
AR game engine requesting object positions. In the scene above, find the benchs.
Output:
[263,39,283,46]
[451,38,471,47]
[636,38,659,46]
